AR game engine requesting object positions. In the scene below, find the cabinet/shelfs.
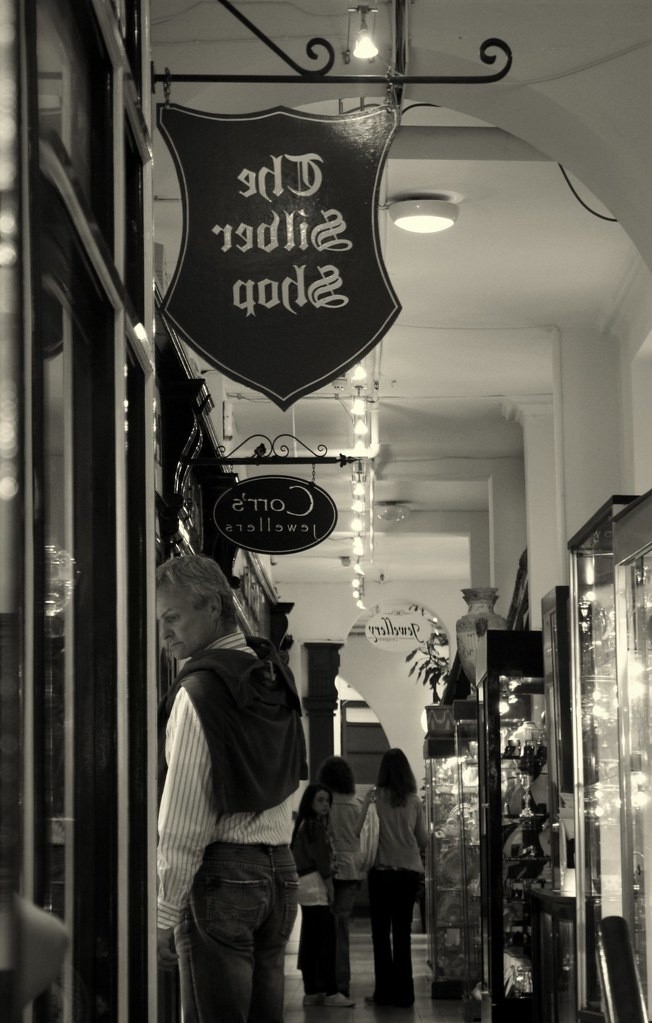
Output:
[448,699,483,1023]
[473,630,558,1023]
[618,485,652,1021]
[422,705,469,1000]
[564,496,644,1022]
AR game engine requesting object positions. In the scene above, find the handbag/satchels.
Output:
[356,786,380,873]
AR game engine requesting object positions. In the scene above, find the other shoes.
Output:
[302,992,356,1007]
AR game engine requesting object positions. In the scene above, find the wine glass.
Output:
[520,774,534,818]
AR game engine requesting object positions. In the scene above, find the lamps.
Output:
[354,435,365,451]
[351,386,367,416]
[352,537,366,611]
[353,361,366,381]
[387,200,459,234]
[351,514,363,530]
[351,495,364,512]
[352,5,379,59]
[354,417,368,435]
[353,481,365,496]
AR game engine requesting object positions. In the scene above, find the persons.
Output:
[311,754,365,1001]
[143,551,312,1023]
[353,747,431,1011]
[290,782,354,1008]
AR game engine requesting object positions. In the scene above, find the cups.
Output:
[507,738,521,757]
